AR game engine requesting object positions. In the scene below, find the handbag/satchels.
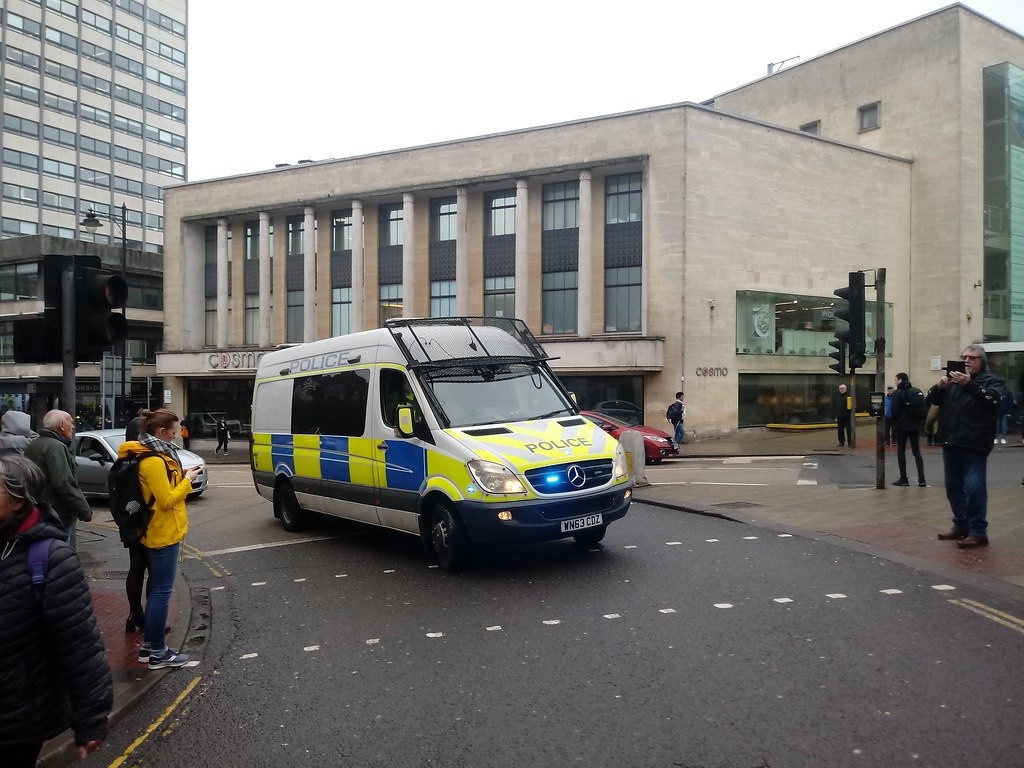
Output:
[180,426,188,437]
[227,430,231,438]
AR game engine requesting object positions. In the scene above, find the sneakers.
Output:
[148,647,192,669]
[138,643,179,663]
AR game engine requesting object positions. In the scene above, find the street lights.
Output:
[78,202,128,429]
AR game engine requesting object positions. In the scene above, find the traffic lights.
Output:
[848,343,867,368]
[833,272,865,353]
[78,266,128,362]
[828,338,846,377]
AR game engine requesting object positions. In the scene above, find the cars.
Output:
[594,399,644,422]
[577,410,680,465]
[189,411,242,438]
[64,428,209,505]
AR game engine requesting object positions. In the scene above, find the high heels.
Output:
[165,626,171,635]
[126,609,146,632]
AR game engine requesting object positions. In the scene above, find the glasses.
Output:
[960,355,984,361]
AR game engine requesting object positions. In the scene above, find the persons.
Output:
[892,373,926,487]
[928,344,1008,548]
[833,384,852,446]
[884,384,941,446]
[0,410,113,768]
[118,409,191,669]
[993,377,1013,444]
[1021,417,1024,487]
[180,414,191,451]
[214,416,230,456]
[670,392,686,449]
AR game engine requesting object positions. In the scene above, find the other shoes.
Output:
[224,453,229,456]
[1000,438,1006,444]
[994,438,998,445]
[848,442,851,447]
[214,448,218,455]
[919,481,926,487]
[938,526,968,539]
[957,536,988,547]
[837,443,844,446]
[892,477,909,486]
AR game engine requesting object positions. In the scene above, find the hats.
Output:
[887,385,894,390]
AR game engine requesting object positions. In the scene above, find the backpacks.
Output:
[667,402,677,419]
[108,450,172,549]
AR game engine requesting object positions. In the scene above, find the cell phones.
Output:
[946,361,966,378]
[193,466,203,472]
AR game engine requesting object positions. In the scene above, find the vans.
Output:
[247,313,635,576]
